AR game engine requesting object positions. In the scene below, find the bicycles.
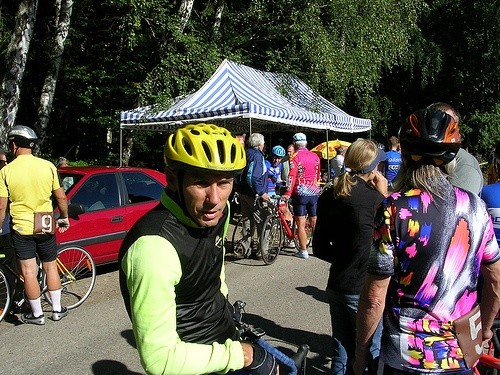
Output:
[231,196,313,265]
[0,245,96,322]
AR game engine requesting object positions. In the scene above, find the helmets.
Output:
[163,123,248,174]
[292,132,306,142]
[272,145,286,157]
[7,125,38,148]
[376,143,386,151]
[398,109,462,169]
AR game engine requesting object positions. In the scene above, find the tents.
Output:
[120,59,373,184]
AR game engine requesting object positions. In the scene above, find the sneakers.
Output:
[295,250,309,258]
[20,311,45,325]
[52,306,69,320]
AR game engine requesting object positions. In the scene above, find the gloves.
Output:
[55,216,70,233]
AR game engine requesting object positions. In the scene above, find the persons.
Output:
[118,123,281,375]
[230,103,500,375]
[1,126,73,325]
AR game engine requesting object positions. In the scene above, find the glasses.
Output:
[8,139,17,146]
[0,160,8,165]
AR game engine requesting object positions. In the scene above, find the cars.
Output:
[0,166,169,274]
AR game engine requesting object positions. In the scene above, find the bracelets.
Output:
[356,338,372,350]
